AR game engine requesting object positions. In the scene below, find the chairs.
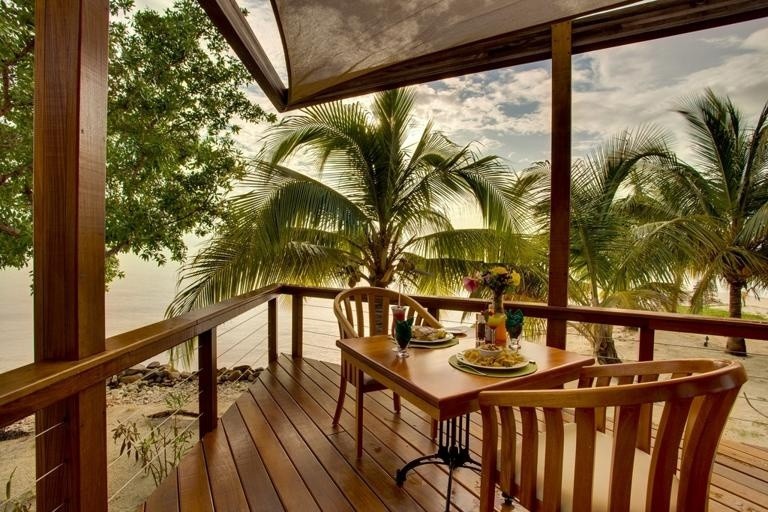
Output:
[475,355,750,512]
[332,286,447,466]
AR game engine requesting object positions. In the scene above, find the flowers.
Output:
[462,266,522,293]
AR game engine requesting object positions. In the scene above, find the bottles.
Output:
[475,313,523,350]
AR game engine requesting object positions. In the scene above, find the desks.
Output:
[335,322,595,512]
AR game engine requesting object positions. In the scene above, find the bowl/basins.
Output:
[478,345,502,357]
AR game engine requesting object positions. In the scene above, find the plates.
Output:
[455,349,530,371]
[411,326,455,345]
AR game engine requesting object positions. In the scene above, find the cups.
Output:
[393,324,412,359]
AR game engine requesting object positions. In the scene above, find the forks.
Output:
[453,360,488,376]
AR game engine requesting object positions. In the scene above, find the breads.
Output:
[411,326,447,340]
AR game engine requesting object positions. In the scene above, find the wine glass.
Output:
[390,305,406,352]
